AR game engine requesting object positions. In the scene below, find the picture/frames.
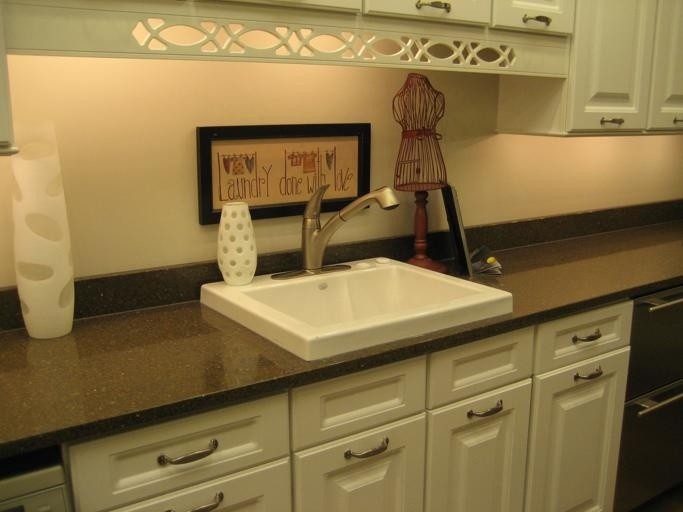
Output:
[195,126,372,224]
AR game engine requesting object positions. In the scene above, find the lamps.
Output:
[391,71,453,274]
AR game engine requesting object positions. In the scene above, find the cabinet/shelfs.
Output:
[493,2,683,140]
[54,387,292,510]
[291,321,539,512]
[526,293,635,510]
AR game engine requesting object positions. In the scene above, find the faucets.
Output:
[301,184,400,271]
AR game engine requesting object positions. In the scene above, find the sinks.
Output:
[199,255,514,361]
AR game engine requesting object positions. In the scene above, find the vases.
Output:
[9,144,76,340]
[217,202,258,286]
